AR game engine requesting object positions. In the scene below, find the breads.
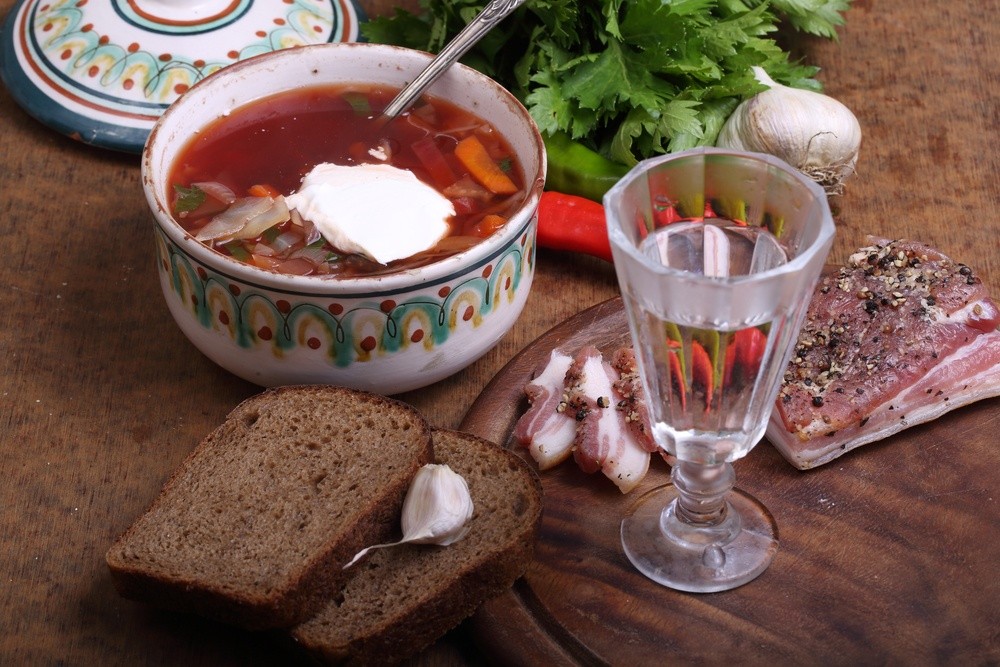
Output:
[105,384,547,661]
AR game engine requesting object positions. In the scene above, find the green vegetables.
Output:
[354,0,851,166]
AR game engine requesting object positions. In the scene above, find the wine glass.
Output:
[602,148,836,592]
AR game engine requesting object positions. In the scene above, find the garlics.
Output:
[339,463,474,571]
[716,60,862,200]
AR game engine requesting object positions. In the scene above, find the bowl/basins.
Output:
[140,43,548,396]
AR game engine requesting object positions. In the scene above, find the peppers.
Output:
[537,128,625,265]
